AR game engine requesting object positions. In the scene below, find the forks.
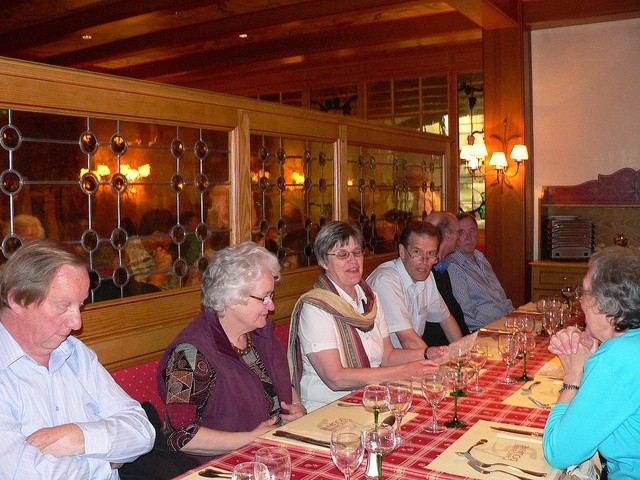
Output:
[465,451,548,477]
[466,461,533,480]
[528,396,552,409]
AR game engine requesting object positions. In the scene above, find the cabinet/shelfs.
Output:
[528,259,590,301]
[538,182,640,260]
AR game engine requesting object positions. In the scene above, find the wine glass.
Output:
[517,335,537,381]
[504,317,525,334]
[386,380,413,446]
[498,335,519,385]
[253,447,292,480]
[536,295,566,335]
[445,371,468,429]
[231,462,269,480]
[469,342,488,394]
[449,347,472,383]
[421,370,448,434]
[519,318,535,333]
[360,422,396,480]
[330,426,364,480]
[362,385,389,432]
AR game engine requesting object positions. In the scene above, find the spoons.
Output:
[455,439,488,457]
[205,468,253,480]
[521,381,541,392]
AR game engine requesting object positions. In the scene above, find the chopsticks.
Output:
[272,430,331,449]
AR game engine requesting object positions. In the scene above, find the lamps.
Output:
[118,164,152,201]
[79,166,110,196]
[490,145,529,194]
[459,143,488,183]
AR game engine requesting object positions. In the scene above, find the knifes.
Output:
[490,426,543,438]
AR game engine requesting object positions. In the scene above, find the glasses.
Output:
[326,248,363,259]
[250,293,274,305]
[403,247,439,260]
[574,286,593,301]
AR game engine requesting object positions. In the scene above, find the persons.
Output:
[280,203,319,267]
[364,219,462,350]
[1,213,46,275]
[1,239,157,480]
[171,212,203,266]
[422,212,471,347]
[447,212,516,334]
[157,241,308,466]
[287,220,449,414]
[542,246,640,480]
[112,208,177,291]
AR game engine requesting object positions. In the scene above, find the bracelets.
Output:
[558,382,579,392]
[423,347,429,359]
